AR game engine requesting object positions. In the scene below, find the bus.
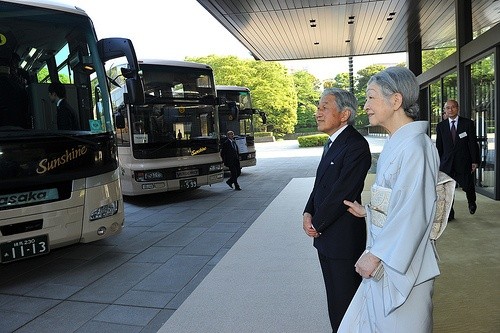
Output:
[91,56,224,200]
[213,85,267,169]
[0,1,146,264]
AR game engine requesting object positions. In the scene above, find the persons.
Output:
[221,131,242,190]
[435,99,479,223]
[302,89,371,333]
[48,83,79,131]
[340,67,457,333]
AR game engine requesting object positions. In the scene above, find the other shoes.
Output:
[235,187,243,190]
[226,181,233,188]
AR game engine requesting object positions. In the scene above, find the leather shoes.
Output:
[448,213,455,221]
[468,203,477,214]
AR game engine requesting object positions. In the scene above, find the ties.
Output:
[451,120,457,137]
[324,138,332,157]
